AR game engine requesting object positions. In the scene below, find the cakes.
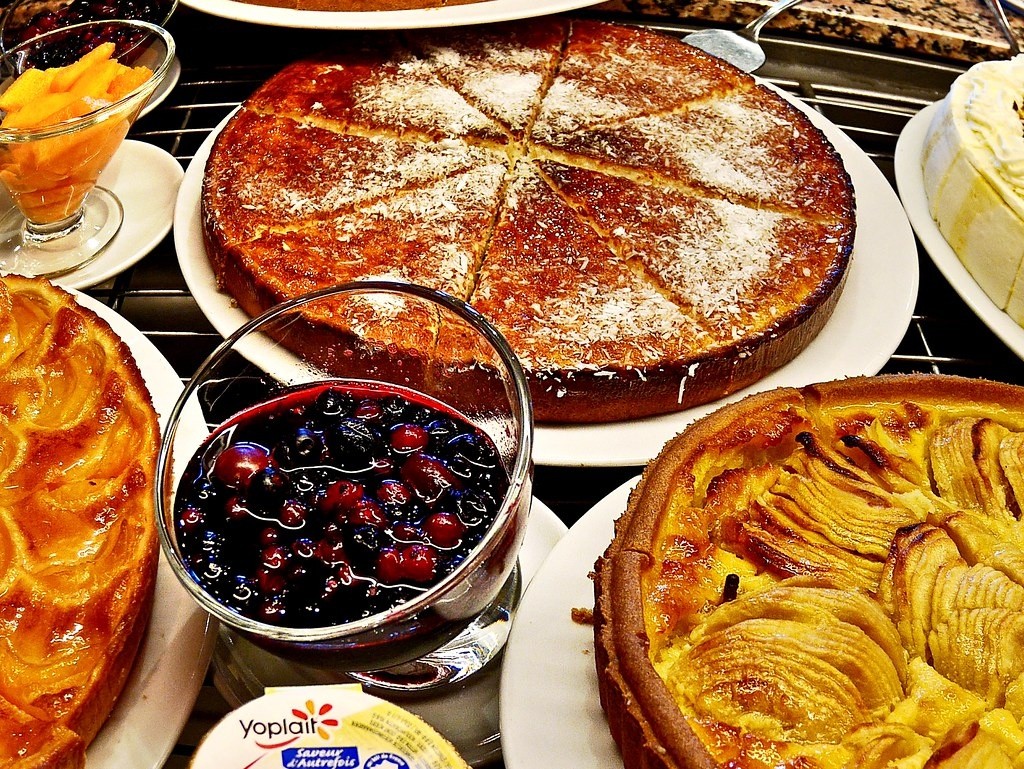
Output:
[0,273,173,769]
[922,50,1024,329]
[587,371,1024,769]
[199,16,858,421]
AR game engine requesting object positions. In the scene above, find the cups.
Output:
[0,38,178,75]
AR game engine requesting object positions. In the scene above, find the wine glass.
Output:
[0,18,177,279]
[153,278,533,693]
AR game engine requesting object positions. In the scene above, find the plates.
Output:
[0,286,220,769]
[894,98,1024,362]
[498,473,642,769]
[173,73,919,467]
[0,140,185,289]
[0,38,180,122]
[213,494,567,763]
